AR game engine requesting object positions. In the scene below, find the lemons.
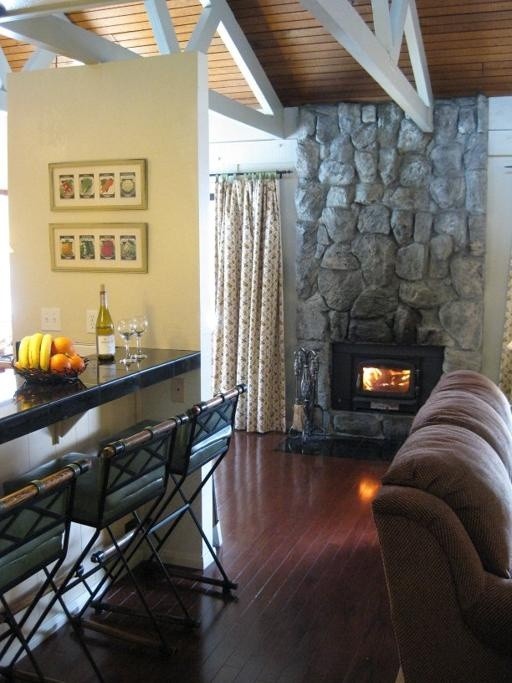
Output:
[49,337,85,373]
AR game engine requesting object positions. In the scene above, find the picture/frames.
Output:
[47,159,147,211]
[50,222,148,274]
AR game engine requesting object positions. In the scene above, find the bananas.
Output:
[18,333,53,371]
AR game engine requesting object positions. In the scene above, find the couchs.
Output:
[370,370,511,683]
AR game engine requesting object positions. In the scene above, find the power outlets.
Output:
[86,308,100,334]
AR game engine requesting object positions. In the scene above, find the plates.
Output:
[60,238,137,261]
[60,175,136,199]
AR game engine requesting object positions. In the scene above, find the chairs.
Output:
[89,382,248,633]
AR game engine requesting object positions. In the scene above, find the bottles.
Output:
[93,283,117,360]
[96,361,118,384]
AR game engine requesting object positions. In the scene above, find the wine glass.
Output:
[118,315,150,364]
